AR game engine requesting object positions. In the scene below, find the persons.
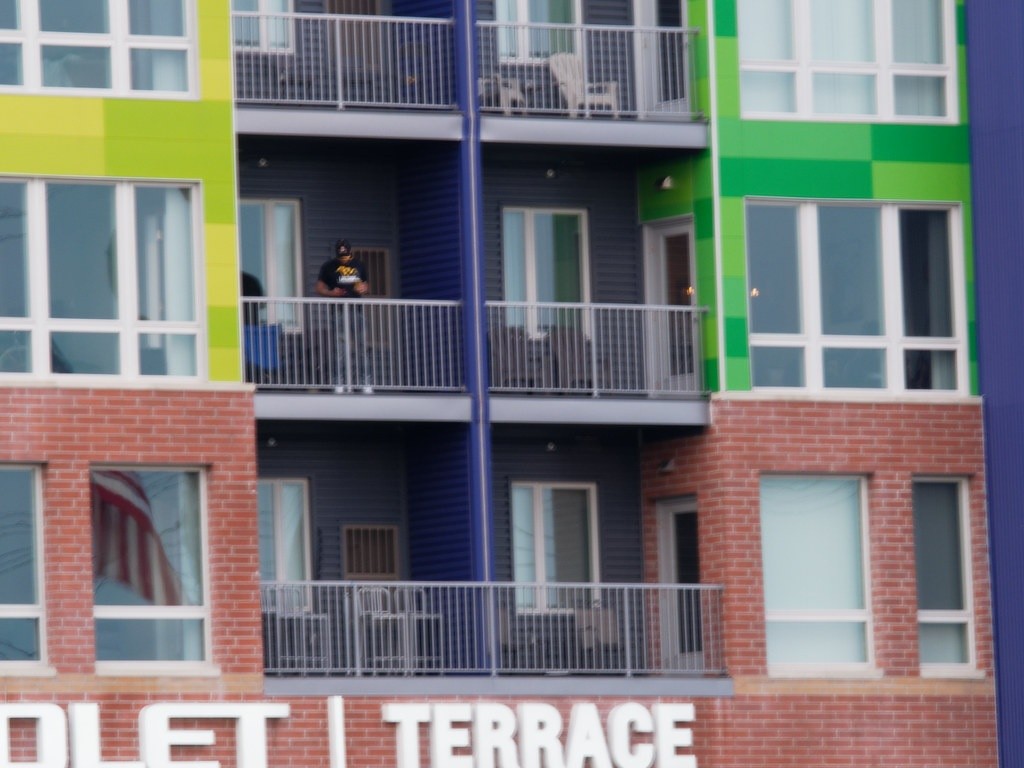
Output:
[318,239,378,393]
[243,272,266,324]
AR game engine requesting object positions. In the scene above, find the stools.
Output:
[262,586,330,676]
[356,587,407,677]
[395,587,444,675]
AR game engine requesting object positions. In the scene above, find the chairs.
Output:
[546,324,613,396]
[546,50,619,118]
[487,324,547,398]
[575,604,643,671]
[497,604,537,669]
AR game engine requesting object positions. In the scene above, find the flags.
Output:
[52,342,182,604]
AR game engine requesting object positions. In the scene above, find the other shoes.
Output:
[335,387,344,393]
[362,386,372,393]
